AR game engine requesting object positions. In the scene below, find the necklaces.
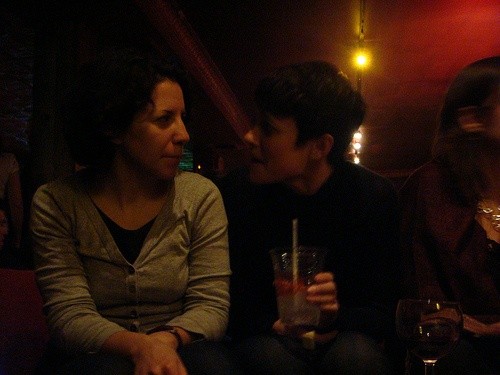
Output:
[479,200,499,235]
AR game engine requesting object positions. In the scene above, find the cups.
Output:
[272,248,322,334]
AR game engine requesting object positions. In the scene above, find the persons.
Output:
[0,152,23,269]
[30,57,231,375]
[210,61,402,374]
[398,55,500,374]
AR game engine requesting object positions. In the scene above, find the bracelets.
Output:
[146,325,183,351]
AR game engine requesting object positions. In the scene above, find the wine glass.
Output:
[396,299,464,375]
